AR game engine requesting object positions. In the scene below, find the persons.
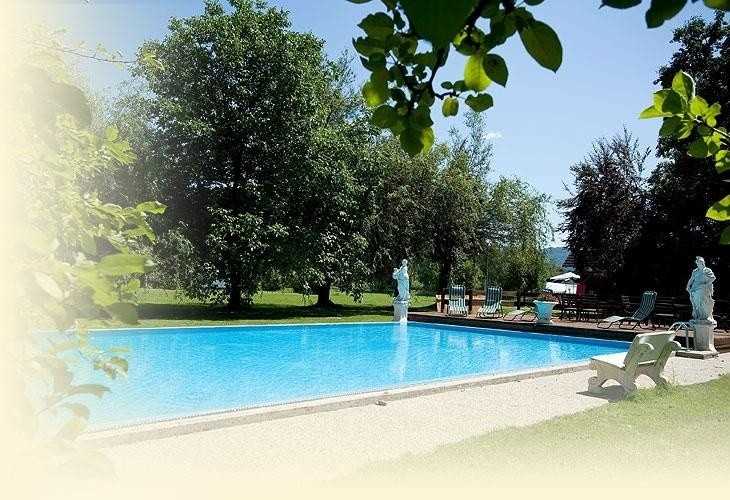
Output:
[684,255,716,320]
[392,259,411,301]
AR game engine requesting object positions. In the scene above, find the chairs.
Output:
[447,284,679,331]
[589,332,682,394]
